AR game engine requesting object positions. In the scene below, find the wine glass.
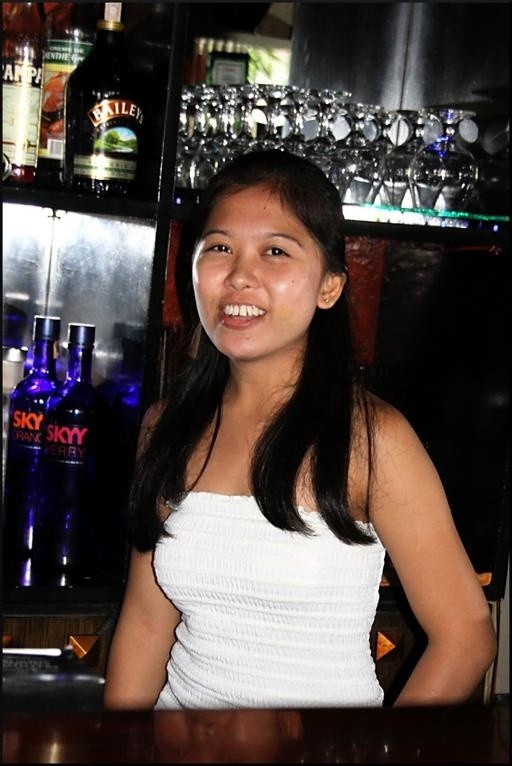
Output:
[179,73,480,214]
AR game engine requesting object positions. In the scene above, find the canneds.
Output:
[41,39,94,184]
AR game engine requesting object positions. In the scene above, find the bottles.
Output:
[37,323,99,589]
[65,0,144,198]
[37,0,98,185]
[2,345,28,509]
[1,0,42,186]
[3,313,55,588]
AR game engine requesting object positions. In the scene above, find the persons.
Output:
[100,148,500,710]
[151,710,305,762]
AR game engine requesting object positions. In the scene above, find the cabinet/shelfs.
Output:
[163,208,510,607]
[1,0,181,643]
